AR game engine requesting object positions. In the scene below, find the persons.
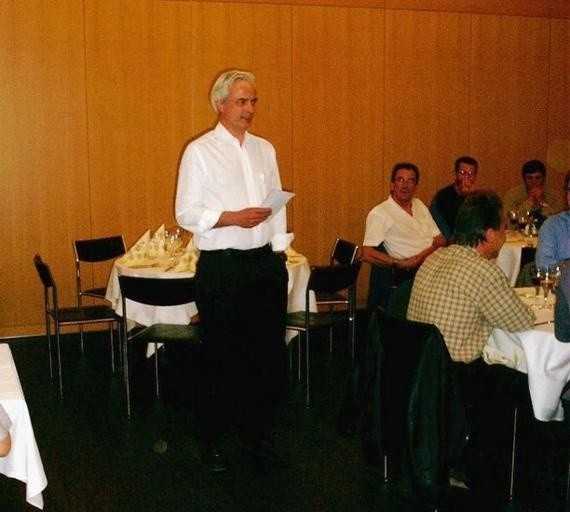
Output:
[358,162,447,309]
[0,403,13,457]
[534,169,570,272]
[554,265,570,439]
[501,160,564,230]
[175,70,295,471]
[407,190,535,365]
[429,156,478,245]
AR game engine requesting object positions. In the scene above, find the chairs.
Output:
[274,238,360,411]
[367,304,461,511]
[33,235,216,415]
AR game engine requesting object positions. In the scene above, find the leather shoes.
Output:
[237,431,288,460]
[190,440,227,471]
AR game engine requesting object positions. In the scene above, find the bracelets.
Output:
[393,258,398,267]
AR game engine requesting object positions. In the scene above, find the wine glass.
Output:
[509,210,541,238]
[528,264,562,308]
